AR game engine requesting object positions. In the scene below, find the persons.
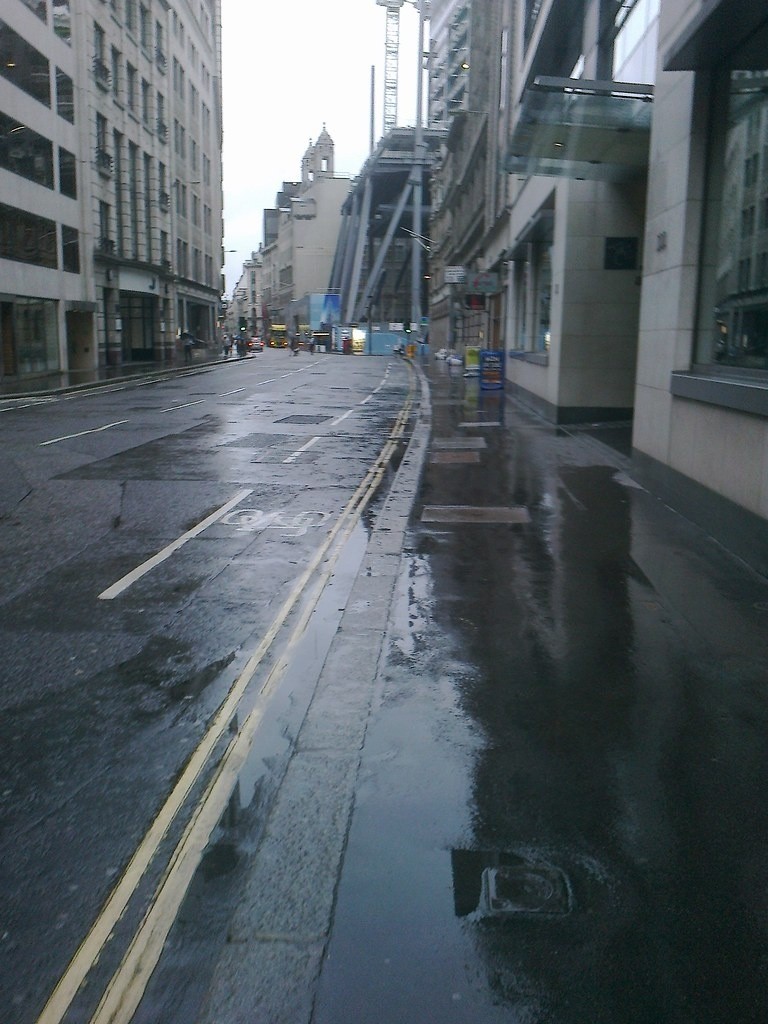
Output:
[222,325,250,358]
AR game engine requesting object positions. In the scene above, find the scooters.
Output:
[238,345,247,358]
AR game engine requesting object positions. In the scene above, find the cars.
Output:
[246,337,264,354]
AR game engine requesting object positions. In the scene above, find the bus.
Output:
[294,324,312,351]
[269,324,288,348]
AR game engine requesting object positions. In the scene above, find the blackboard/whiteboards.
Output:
[478,350,505,390]
